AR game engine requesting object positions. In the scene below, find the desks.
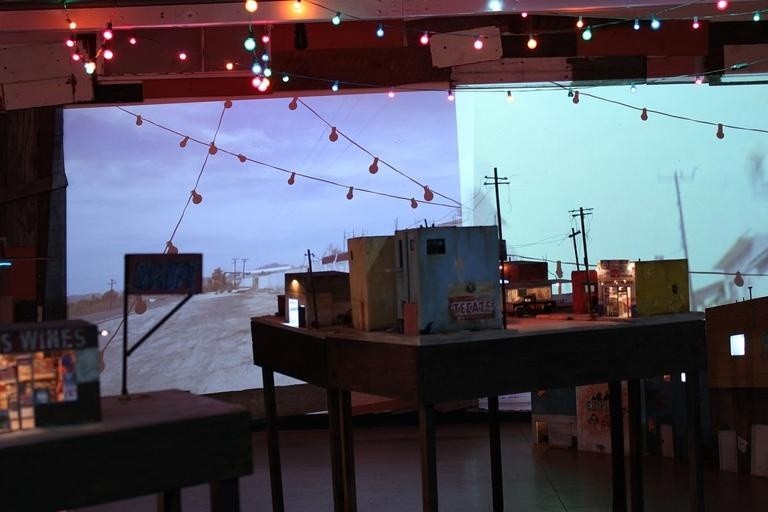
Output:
[0,388,253,512]
[251,312,711,512]
